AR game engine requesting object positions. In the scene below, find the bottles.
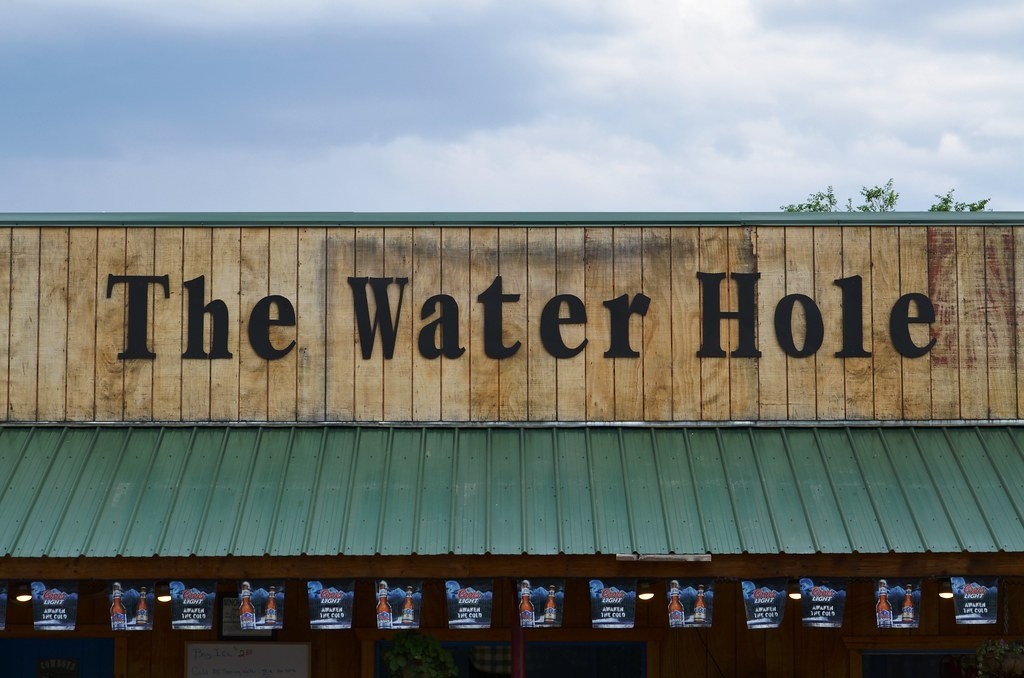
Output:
[519,583,535,628]
[238,584,256,630]
[109,585,126,631]
[376,584,393,629]
[876,582,893,628]
[694,585,706,623]
[401,585,415,624]
[667,582,684,627]
[544,586,555,623]
[901,584,914,623]
[265,585,277,625]
[136,587,148,625]
